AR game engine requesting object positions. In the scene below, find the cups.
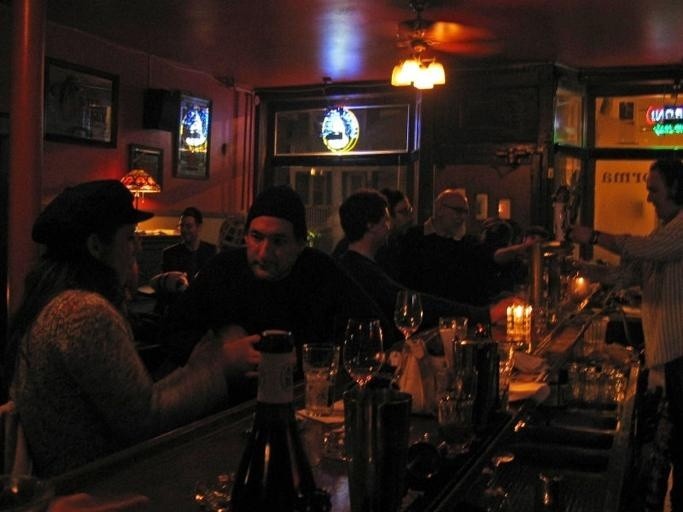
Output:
[493,453,514,488]
[537,470,572,509]
[566,358,628,400]
[436,369,473,429]
[1,473,53,512]
[583,313,608,344]
[299,346,334,415]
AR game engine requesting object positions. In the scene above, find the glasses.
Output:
[177,223,191,230]
[397,201,416,216]
[444,205,469,214]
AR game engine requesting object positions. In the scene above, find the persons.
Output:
[327,184,557,343]
[182,105,203,138]
[151,181,398,403]
[1,176,261,482]
[562,154,681,511]
[153,203,217,317]
[215,211,246,255]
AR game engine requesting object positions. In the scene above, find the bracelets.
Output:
[588,230,601,245]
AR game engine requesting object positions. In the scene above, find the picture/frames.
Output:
[130,142,165,193]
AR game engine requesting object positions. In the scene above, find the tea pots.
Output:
[256,329,298,408]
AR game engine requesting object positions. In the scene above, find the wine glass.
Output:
[396,292,423,373]
[342,317,383,432]
[440,316,469,375]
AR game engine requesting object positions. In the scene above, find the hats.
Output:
[31,180,153,245]
[244,189,305,240]
[218,215,246,249]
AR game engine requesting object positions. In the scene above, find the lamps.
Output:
[389,40,446,91]
[120,168,160,209]
